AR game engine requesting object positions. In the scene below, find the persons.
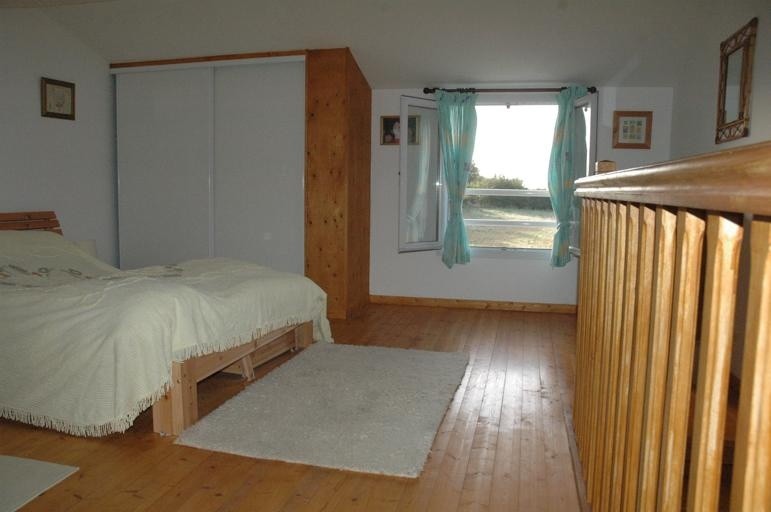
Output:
[393,120,414,142]
[383,132,400,144]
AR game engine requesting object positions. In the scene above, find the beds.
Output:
[0,211,315,437]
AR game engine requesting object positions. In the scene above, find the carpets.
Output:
[0,453,80,512]
[171,340,475,480]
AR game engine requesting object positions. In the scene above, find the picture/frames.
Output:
[612,109,653,150]
[716,15,759,144]
[40,77,76,121]
[379,114,422,147]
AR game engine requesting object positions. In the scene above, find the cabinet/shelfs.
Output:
[109,47,372,321]
